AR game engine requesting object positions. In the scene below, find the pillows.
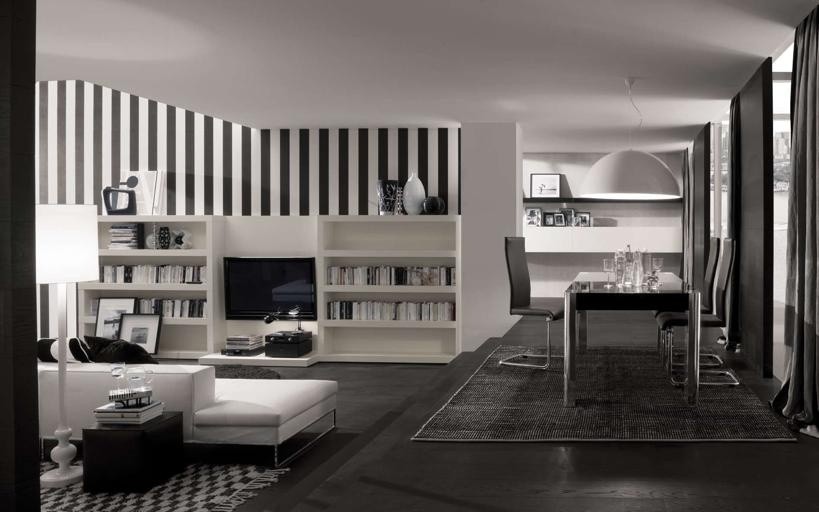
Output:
[37,333,158,364]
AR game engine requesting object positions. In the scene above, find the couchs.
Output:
[38,360,337,470]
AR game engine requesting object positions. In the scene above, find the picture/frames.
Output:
[524,204,590,229]
[116,311,163,355]
[529,170,564,201]
[94,295,137,340]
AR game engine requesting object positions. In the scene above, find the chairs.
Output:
[651,236,725,369]
[655,234,742,388]
[496,234,566,371]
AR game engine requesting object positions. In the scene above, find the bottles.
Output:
[402,172,425,215]
[421,196,446,215]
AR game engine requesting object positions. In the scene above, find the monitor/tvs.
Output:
[222,257,317,321]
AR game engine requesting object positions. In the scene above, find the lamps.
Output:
[34,200,106,488]
[572,75,684,202]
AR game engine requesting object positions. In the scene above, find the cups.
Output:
[375,180,402,215]
[567,215,580,226]
[127,366,154,391]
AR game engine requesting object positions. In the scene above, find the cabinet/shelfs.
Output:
[264,330,313,358]
[75,214,226,360]
[79,412,185,495]
[523,197,683,253]
[315,213,464,365]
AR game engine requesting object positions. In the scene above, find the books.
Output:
[326,264,456,321]
[225,334,263,350]
[93,387,164,425]
[101,224,207,318]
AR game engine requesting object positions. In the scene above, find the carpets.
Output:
[409,343,800,445]
[39,459,291,511]
[199,363,281,381]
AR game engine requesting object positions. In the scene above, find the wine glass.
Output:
[603,245,663,292]
[109,361,126,393]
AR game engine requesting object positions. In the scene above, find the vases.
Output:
[401,169,427,215]
[375,179,399,216]
